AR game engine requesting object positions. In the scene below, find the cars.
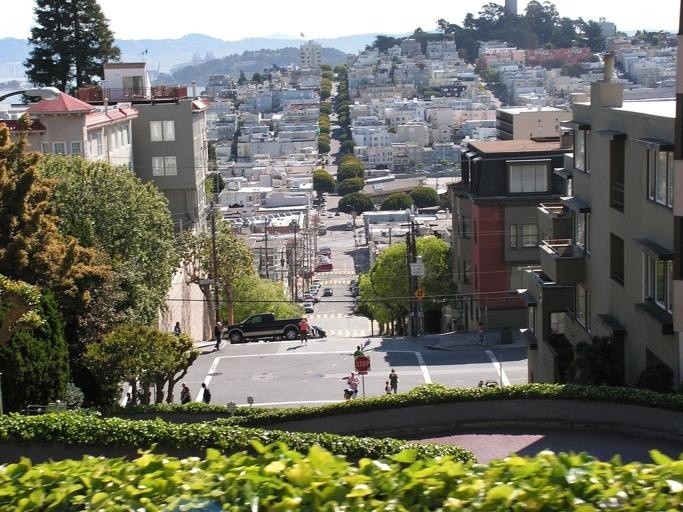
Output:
[307,324,326,338]
[320,193,328,216]
[346,225,353,232]
[300,279,323,314]
[335,212,340,216]
[328,214,335,219]
[350,279,364,315]
[323,288,334,297]
[319,246,333,266]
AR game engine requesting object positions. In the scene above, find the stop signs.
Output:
[355,357,371,372]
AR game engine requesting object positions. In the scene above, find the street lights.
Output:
[346,204,357,236]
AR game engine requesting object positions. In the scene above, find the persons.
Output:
[298,318,309,346]
[125,383,190,406]
[173,321,181,337]
[201,382,210,405]
[388,369,399,394]
[347,372,360,399]
[353,345,363,356]
[213,321,222,351]
[385,380,391,394]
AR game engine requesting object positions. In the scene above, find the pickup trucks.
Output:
[224,313,310,345]
